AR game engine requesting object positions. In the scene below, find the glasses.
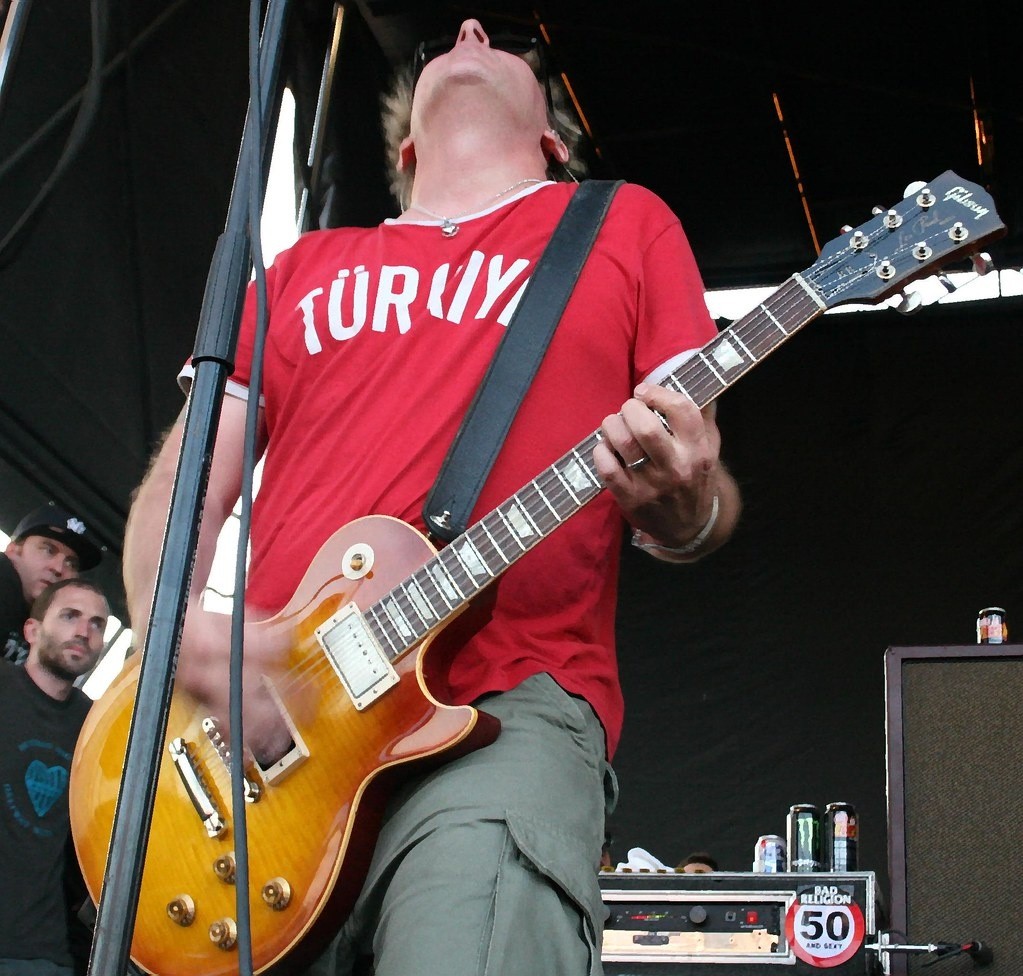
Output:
[411,33,553,117]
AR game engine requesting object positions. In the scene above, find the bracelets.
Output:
[632,496,720,553]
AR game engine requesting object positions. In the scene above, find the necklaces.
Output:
[409,179,541,237]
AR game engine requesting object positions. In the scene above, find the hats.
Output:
[11,508,102,571]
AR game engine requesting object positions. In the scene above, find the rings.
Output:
[627,457,649,470]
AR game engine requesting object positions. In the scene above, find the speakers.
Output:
[881,643,1023,976]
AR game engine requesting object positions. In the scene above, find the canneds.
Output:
[785,804,821,872]
[822,801,858,873]
[976,607,1008,645]
[752,835,787,873]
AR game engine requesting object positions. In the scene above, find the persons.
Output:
[123,17,741,976]
[0,506,112,976]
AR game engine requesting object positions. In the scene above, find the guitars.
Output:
[62,163,1010,976]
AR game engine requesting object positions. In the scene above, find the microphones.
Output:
[967,941,981,953]
